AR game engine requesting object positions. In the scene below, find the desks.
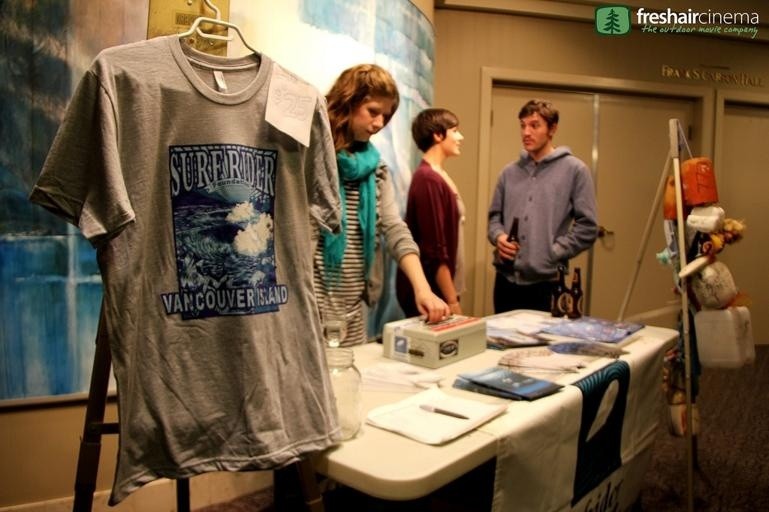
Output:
[304,308,681,512]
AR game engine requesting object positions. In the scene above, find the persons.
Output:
[396,107,464,318]
[489,100,601,314]
[275,64,448,512]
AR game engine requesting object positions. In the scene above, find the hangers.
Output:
[179,1,261,70]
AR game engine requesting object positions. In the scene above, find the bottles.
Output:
[326,347,364,444]
[567,267,584,319]
[551,264,568,319]
[500,217,520,277]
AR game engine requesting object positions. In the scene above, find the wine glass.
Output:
[323,296,347,347]
